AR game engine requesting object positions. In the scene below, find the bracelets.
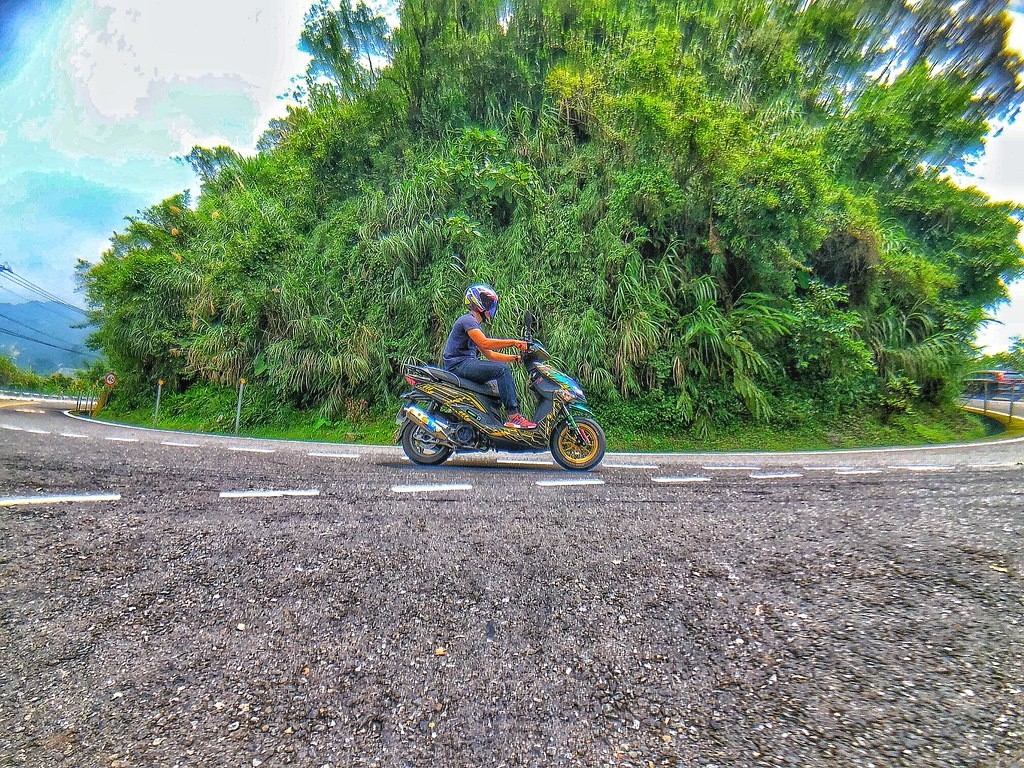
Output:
[513,355,518,361]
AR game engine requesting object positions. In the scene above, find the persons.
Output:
[442,285,539,429]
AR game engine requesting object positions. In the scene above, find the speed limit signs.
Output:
[105,373,118,385]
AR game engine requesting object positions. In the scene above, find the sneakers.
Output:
[504,413,537,430]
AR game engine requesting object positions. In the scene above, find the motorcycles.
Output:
[396,311,606,474]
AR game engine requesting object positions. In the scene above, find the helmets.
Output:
[463,283,499,325]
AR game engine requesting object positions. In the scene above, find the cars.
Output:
[960,371,1024,401]
